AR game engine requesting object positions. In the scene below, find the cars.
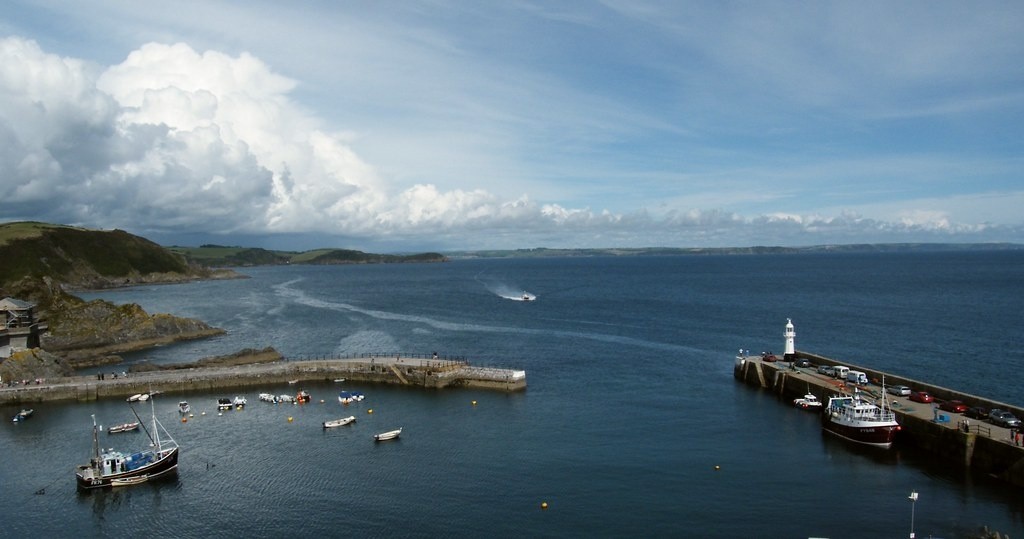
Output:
[966,406,987,419]
[763,354,779,363]
[887,384,911,397]
[909,391,935,404]
[990,411,1022,427]
[939,400,969,412]
[797,358,810,368]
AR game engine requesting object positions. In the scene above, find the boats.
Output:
[178,400,189,414]
[74,379,183,488]
[217,398,233,409]
[11,409,34,422]
[373,426,404,441]
[826,370,901,451]
[258,387,312,403]
[793,391,823,410]
[337,389,367,404]
[108,422,140,433]
[323,416,356,428]
[138,394,149,400]
[126,394,141,402]
[234,396,248,405]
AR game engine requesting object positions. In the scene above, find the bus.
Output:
[818,365,868,386]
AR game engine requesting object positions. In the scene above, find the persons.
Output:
[7,377,45,387]
[433,351,439,361]
[371,355,375,363]
[789,362,795,371]
[112,369,128,380]
[1010,429,1020,446]
[97,370,104,380]
[396,352,400,362]
[962,419,970,433]
